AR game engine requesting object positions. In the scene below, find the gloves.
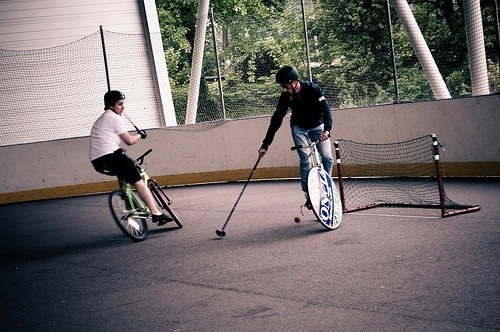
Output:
[138,130,147,139]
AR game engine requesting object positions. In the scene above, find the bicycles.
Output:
[104,149,183,240]
[290,134,343,229]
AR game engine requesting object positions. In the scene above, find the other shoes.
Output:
[126,199,132,210]
[306,199,312,210]
[152,213,173,222]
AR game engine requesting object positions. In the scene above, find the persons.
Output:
[257,65,333,206]
[89,90,173,223]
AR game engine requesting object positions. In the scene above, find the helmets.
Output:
[104,90,125,106]
[276,66,299,84]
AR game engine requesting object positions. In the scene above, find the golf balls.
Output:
[214,154,263,238]
[295,216,299,223]
